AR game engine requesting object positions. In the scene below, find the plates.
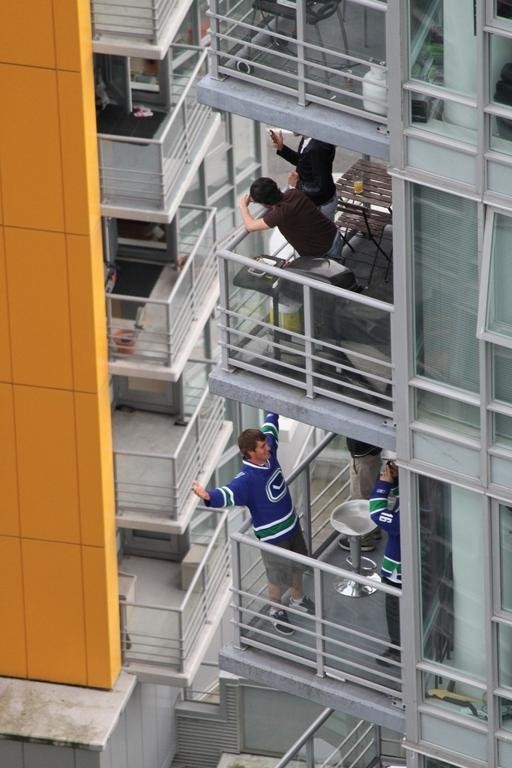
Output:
[248,258,278,277]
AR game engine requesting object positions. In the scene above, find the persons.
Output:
[239,177,343,369]
[337,436,383,552]
[266,128,337,222]
[192,410,319,635]
[368,459,401,667]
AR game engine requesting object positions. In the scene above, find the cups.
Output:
[343,70,354,91]
[352,172,363,194]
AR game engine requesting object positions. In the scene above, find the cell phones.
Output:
[270,129,278,143]
[387,461,391,466]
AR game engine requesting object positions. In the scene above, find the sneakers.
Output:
[270,608,294,635]
[290,596,315,613]
[338,531,382,551]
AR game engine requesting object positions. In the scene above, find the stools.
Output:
[331,500,380,597]
[280,257,366,392]
[244,1,355,100]
[236,253,289,373]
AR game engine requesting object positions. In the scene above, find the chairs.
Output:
[335,202,392,285]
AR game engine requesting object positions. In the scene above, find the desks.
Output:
[336,158,394,281]
